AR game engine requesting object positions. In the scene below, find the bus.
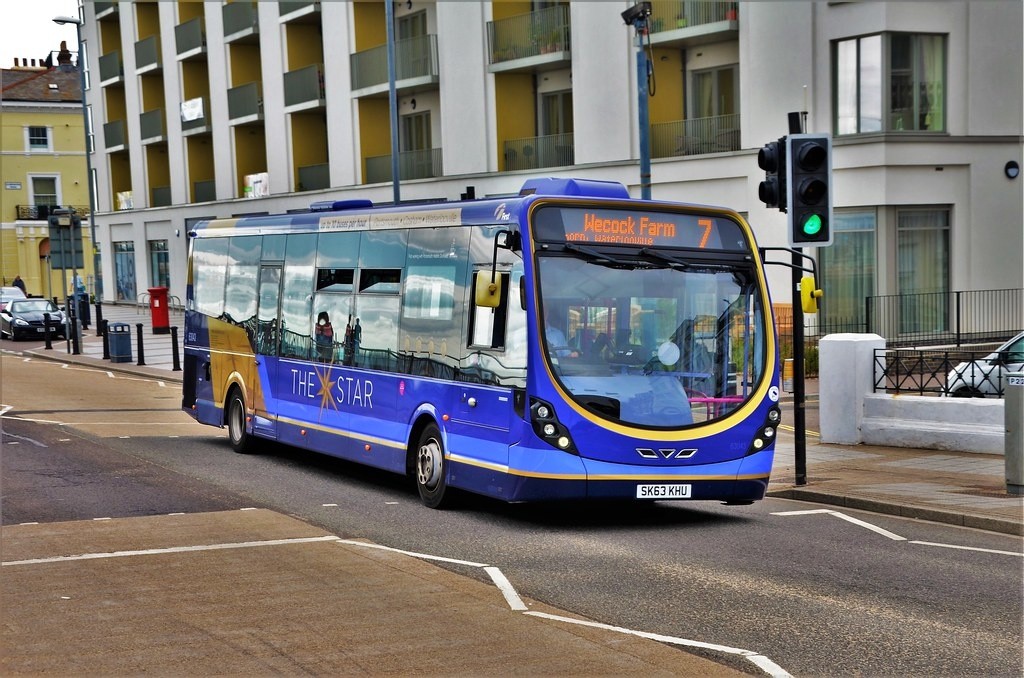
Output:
[179,175,826,517]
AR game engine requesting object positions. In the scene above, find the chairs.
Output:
[677,135,702,154]
[712,130,740,152]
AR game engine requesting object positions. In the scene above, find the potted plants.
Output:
[722,3,736,20]
[554,28,567,52]
[503,44,519,59]
[543,32,556,54]
[531,29,547,54]
[674,15,686,28]
[654,17,663,32]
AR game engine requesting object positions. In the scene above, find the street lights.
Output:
[51,16,104,307]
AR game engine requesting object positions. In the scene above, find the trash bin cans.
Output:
[71,289,91,330]
[107,321,134,364]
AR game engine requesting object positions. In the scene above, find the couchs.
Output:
[555,145,574,166]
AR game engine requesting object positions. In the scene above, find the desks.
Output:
[694,142,716,152]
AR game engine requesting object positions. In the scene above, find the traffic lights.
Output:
[787,132,834,249]
[758,139,785,210]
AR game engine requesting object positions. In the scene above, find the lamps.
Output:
[48,82,59,90]
[1004,160,1019,180]
[661,55,668,60]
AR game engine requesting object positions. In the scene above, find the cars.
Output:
[938,330,1024,397]
[0,298,73,342]
[0,285,29,313]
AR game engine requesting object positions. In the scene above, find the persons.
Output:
[543,300,579,359]
[314,310,362,367]
[12,275,27,296]
[70,270,86,294]
